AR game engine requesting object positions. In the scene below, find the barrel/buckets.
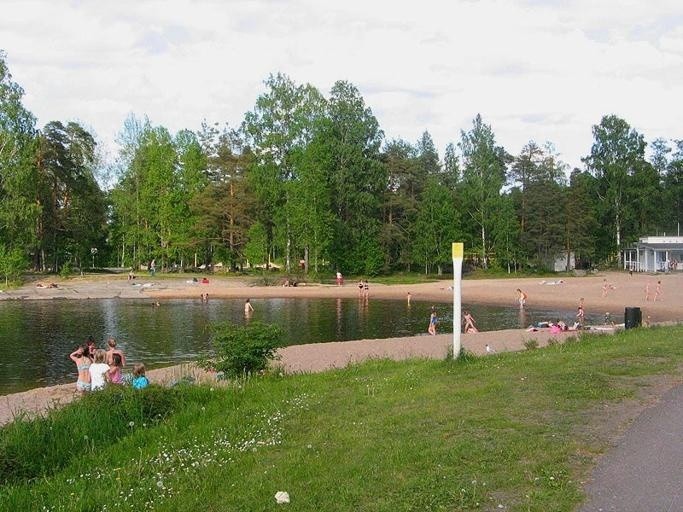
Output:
[624,307,642,332]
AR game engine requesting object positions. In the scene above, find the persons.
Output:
[427,307,437,335]
[516,288,529,306]
[406,291,412,305]
[462,310,479,333]
[601,278,607,297]
[357,279,363,294]
[525,312,614,332]
[69,336,149,398]
[644,282,651,301]
[576,297,584,317]
[335,270,342,285]
[244,297,254,312]
[652,280,664,303]
[364,279,369,294]
[538,278,564,285]
